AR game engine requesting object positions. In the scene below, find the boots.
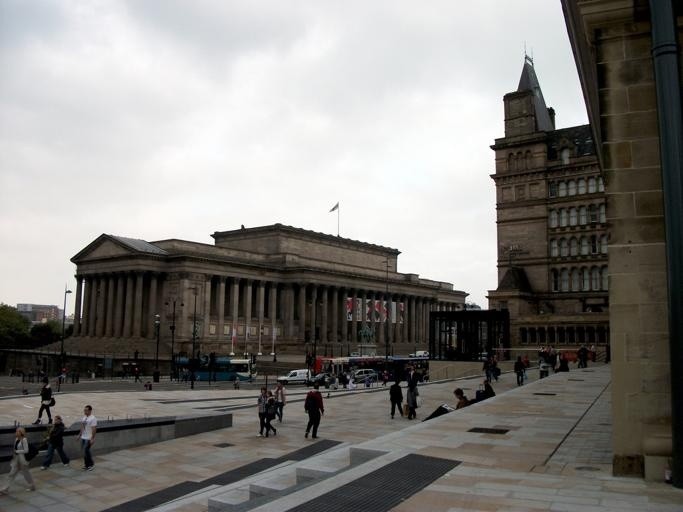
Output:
[32,419,40,425]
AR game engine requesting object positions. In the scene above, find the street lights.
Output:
[58,290,73,390]
[164,299,184,360]
[153,312,162,384]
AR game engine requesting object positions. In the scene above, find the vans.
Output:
[277,369,311,385]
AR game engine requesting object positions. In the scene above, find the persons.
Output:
[324,369,389,391]
[56,367,240,391]
[77,404,97,471]
[33,376,52,425]
[1,425,37,493]
[453,342,597,410]
[390,365,420,420]
[255,380,285,438]
[39,416,70,471]
[304,384,325,439]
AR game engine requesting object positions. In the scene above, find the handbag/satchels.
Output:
[403,405,409,415]
[49,398,55,406]
[416,397,423,407]
[25,445,38,462]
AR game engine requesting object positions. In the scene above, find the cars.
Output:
[478,353,486,360]
[348,369,377,381]
[408,351,429,357]
[307,373,334,386]
[350,352,368,357]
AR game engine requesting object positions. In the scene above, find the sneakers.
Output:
[40,464,96,472]
[0,489,8,495]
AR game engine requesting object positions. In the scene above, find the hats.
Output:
[40,377,48,383]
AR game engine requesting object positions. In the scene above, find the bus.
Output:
[174,356,256,383]
[321,354,430,382]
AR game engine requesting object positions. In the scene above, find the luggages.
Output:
[476,384,485,401]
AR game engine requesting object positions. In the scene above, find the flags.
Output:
[329,203,339,212]
